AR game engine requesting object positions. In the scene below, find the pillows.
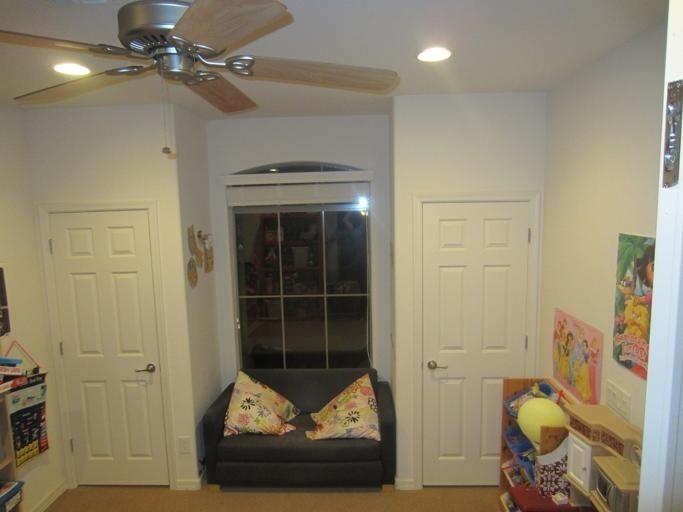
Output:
[305,372,383,442]
[223,370,300,438]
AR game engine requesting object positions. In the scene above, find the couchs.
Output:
[203,368,395,486]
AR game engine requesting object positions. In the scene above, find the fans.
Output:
[0,0,400,157]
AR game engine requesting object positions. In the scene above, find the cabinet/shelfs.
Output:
[498,377,573,512]
[568,432,605,492]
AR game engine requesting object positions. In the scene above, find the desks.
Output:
[508,486,594,512]
